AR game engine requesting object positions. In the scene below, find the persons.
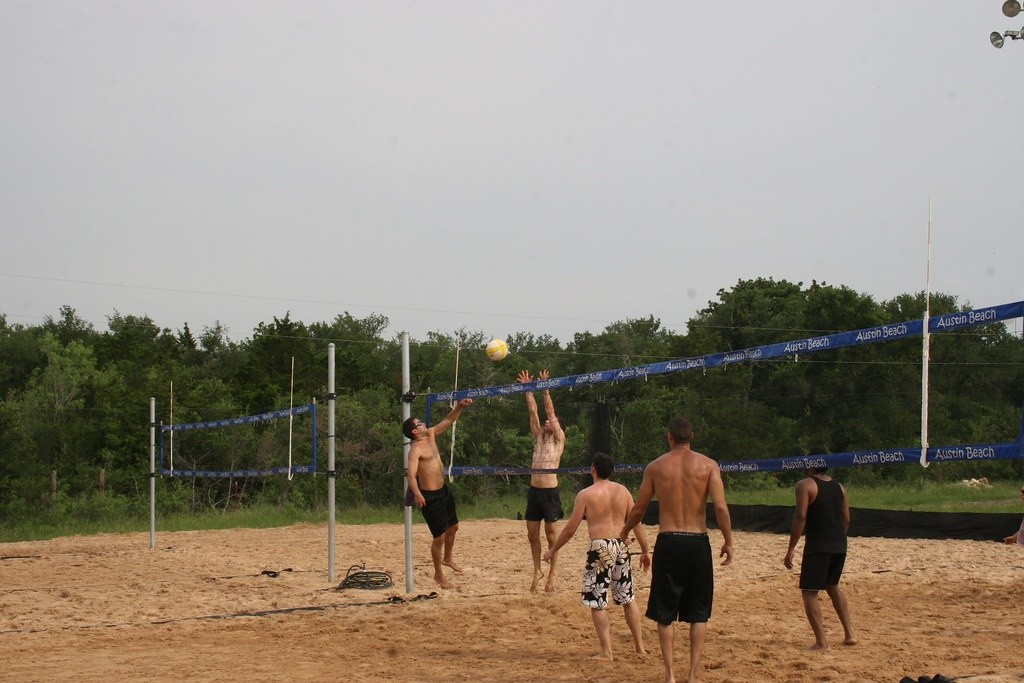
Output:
[403,398,472,588]
[621,416,733,683]
[543,453,651,660]
[784,446,858,648]
[517,369,566,592]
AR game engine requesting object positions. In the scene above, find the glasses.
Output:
[415,421,422,428]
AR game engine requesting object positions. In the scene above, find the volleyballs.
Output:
[487,337,509,362]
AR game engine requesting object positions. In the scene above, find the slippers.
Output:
[410,592,438,602]
[388,596,406,604]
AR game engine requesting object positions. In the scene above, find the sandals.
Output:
[899,673,956,682]
